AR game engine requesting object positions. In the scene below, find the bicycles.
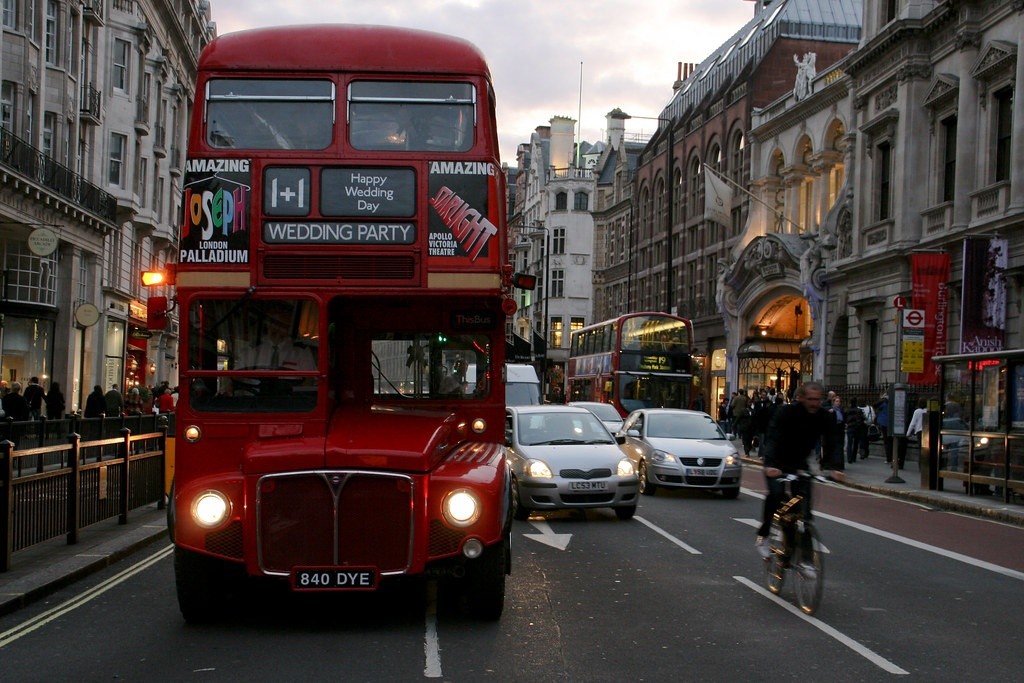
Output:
[761,469,840,615]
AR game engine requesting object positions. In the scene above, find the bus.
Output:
[139,21,538,625]
[565,310,698,421]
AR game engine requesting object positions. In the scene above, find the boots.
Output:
[865,448,869,458]
[859,448,866,460]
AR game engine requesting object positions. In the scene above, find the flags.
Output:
[704,165,732,227]
[503,329,547,359]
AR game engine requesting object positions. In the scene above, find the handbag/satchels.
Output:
[151,404,159,413]
[867,424,880,441]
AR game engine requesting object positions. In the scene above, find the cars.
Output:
[614,409,744,498]
[566,401,624,438]
[506,405,643,522]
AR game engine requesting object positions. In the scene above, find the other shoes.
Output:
[755,536,770,558]
[796,562,816,580]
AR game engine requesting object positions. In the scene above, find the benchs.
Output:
[971,459,1024,472]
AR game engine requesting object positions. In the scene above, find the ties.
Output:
[270,346,279,370]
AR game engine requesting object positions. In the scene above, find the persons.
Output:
[655,333,680,351]
[716,386,965,472]
[215,305,331,410]
[543,386,567,405]
[754,379,844,577]
[620,388,635,399]
[0,372,179,468]
[792,53,812,102]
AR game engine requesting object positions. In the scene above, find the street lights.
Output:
[612,112,675,316]
[512,224,550,394]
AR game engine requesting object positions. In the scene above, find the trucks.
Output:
[462,364,542,405]
